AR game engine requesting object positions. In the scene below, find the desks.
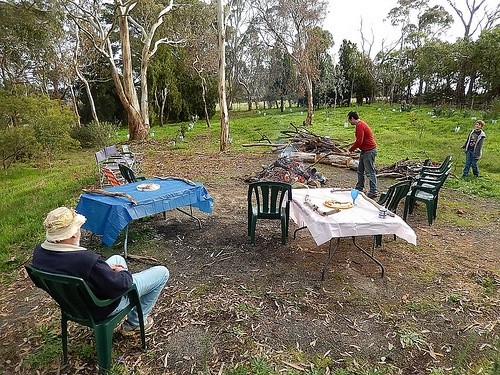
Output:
[74,177,214,259]
[281,185,416,281]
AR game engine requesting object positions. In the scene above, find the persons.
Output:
[347,112,377,198]
[33,207,169,336]
[462,121,486,177]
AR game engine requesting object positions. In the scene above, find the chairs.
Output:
[22,264,148,375]
[374,155,454,250]
[246,181,292,246]
[94,144,147,189]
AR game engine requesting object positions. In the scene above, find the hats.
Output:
[43,204,87,243]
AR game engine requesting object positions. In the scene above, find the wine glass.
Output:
[350,189,359,206]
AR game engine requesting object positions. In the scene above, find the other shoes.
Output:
[368,193,377,199]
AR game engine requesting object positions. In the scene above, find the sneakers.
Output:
[117,317,155,336]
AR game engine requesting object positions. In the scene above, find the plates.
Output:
[136,183,160,192]
[323,199,354,208]
